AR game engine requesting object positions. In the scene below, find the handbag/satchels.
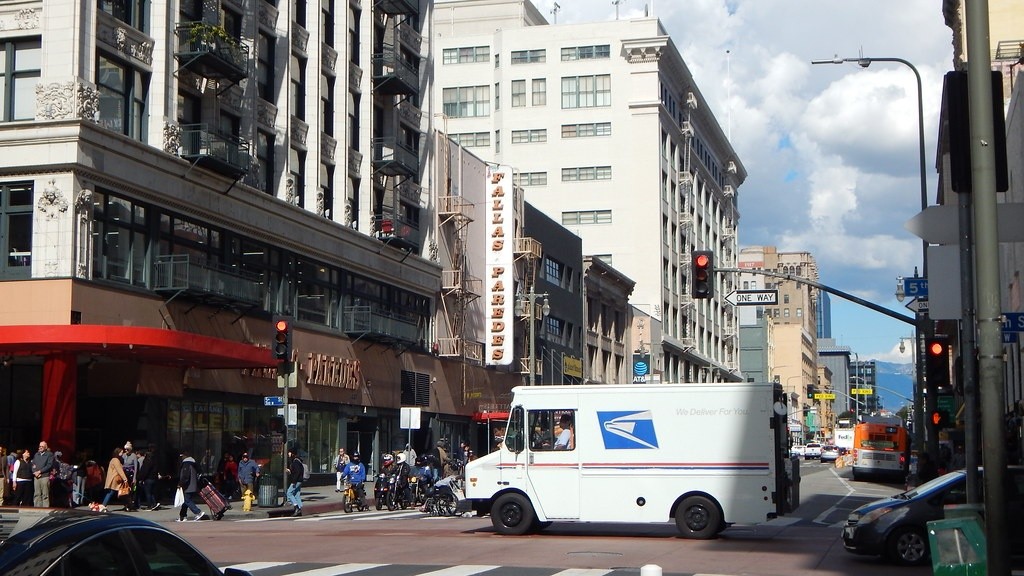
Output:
[174,487,185,508]
[118,483,132,499]
[337,465,346,472]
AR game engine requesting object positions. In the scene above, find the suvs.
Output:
[805,443,822,461]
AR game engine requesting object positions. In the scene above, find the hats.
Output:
[124,441,132,450]
[180,452,191,457]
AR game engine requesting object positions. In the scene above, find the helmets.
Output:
[289,447,297,454]
[351,451,360,464]
[415,457,422,466]
[397,453,406,464]
[384,454,394,467]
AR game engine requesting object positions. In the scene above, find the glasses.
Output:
[243,455,248,458]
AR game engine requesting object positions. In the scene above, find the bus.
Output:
[852,417,914,483]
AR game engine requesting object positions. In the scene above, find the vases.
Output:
[382,220,392,232]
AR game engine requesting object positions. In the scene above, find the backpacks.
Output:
[293,458,310,481]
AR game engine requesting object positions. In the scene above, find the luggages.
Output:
[197,480,231,520]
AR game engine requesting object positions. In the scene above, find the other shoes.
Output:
[193,512,205,522]
[411,504,415,509]
[176,517,187,522]
[420,508,429,512]
[124,503,160,512]
[335,489,340,492]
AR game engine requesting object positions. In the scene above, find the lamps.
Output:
[4,352,14,365]
[88,356,99,369]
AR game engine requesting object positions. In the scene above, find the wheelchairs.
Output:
[424,484,459,517]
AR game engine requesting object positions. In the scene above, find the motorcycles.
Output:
[340,478,366,514]
[371,469,441,511]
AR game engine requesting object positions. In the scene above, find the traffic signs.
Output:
[726,289,780,306]
[905,297,929,314]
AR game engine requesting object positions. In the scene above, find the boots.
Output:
[298,509,302,516]
[292,505,300,516]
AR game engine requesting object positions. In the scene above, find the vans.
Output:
[456,383,797,539]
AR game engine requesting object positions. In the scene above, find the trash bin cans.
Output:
[257,473,279,507]
[926,515,988,576]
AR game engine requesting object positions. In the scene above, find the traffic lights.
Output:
[807,383,814,399]
[271,315,292,361]
[691,250,715,300]
[931,410,954,430]
[923,337,950,395]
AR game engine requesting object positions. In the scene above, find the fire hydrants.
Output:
[240,489,256,511]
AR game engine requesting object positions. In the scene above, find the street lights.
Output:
[849,376,866,413]
[813,57,926,481]
[898,329,917,404]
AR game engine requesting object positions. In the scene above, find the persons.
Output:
[176,450,206,522]
[217,452,238,499]
[237,453,261,512]
[102,441,160,511]
[333,448,350,492]
[917,447,951,484]
[285,448,304,516]
[200,449,217,477]
[0,441,88,509]
[554,414,573,451]
[376,440,476,511]
[340,451,366,501]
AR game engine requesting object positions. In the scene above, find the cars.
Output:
[819,444,842,463]
[2,515,253,576]
[844,463,1024,567]
[791,445,805,456]
